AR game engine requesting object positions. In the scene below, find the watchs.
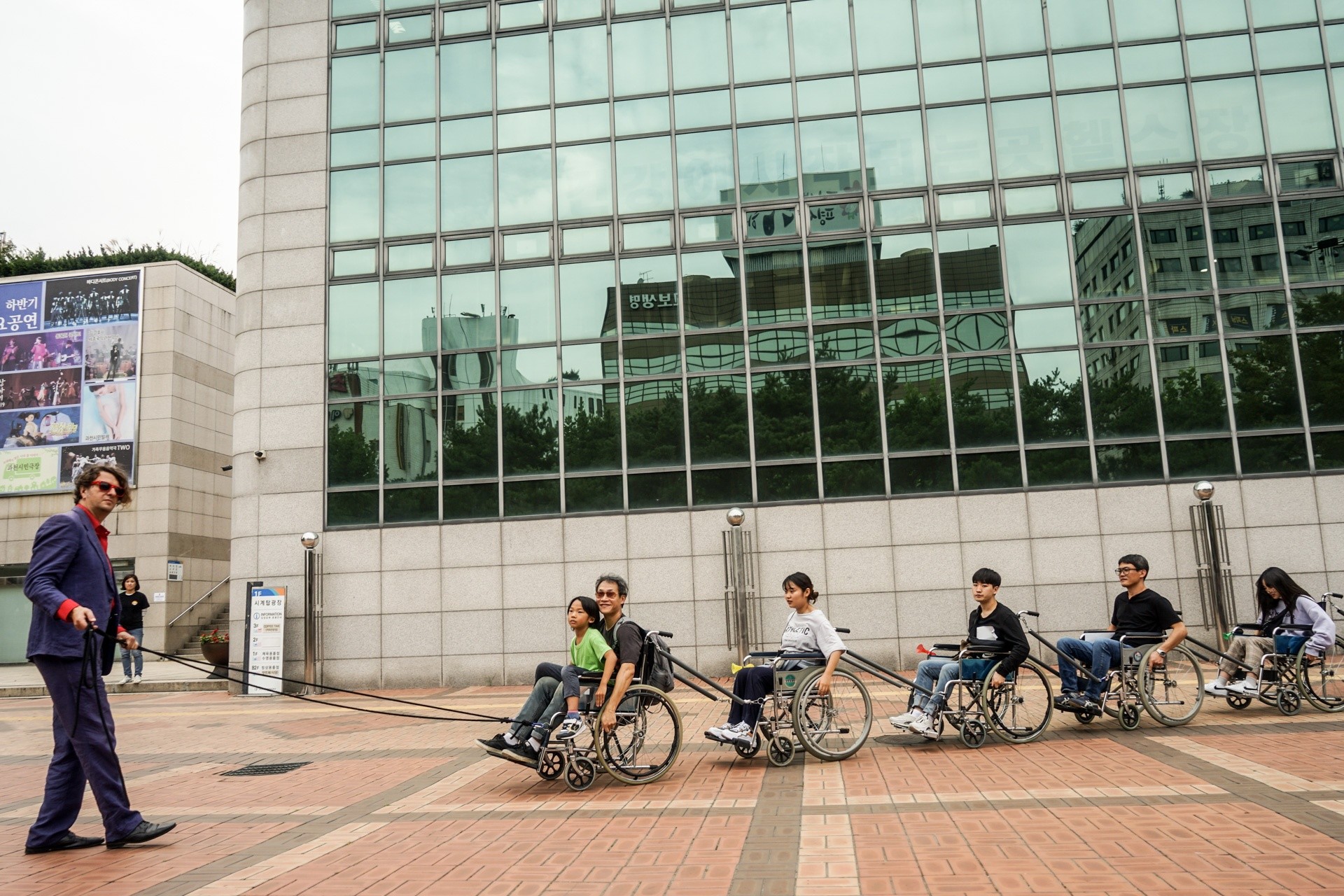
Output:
[1156,649,1167,658]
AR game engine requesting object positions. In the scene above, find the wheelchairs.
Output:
[1207,592,1344,715]
[705,627,873,766]
[1053,611,1205,730]
[486,629,683,791]
[894,610,1053,748]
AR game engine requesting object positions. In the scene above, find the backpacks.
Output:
[613,616,674,715]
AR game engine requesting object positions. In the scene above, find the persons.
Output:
[0,333,136,485]
[533,596,619,740]
[1196,567,1336,697]
[116,574,150,685]
[1052,554,1187,710]
[476,574,646,762]
[704,572,848,748]
[50,284,132,327]
[24,466,176,855]
[890,567,1030,733]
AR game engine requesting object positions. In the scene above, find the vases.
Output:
[201,644,229,679]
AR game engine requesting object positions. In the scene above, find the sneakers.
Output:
[1068,695,1103,711]
[1047,692,1081,708]
[721,719,757,747]
[1225,680,1258,695]
[475,732,523,754]
[502,740,542,766]
[909,712,935,733]
[1196,679,1228,696]
[555,715,588,740]
[889,708,923,731]
[705,722,734,743]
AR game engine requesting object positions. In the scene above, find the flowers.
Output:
[199,629,230,644]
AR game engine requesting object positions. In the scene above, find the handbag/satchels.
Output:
[967,636,1010,660]
[1262,608,1288,639]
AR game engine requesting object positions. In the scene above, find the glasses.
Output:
[1115,567,1143,576]
[595,591,619,599]
[90,481,126,497]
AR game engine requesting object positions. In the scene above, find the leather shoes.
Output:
[25,831,104,855]
[106,820,177,850]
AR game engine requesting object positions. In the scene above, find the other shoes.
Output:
[133,675,142,683]
[118,674,132,685]
